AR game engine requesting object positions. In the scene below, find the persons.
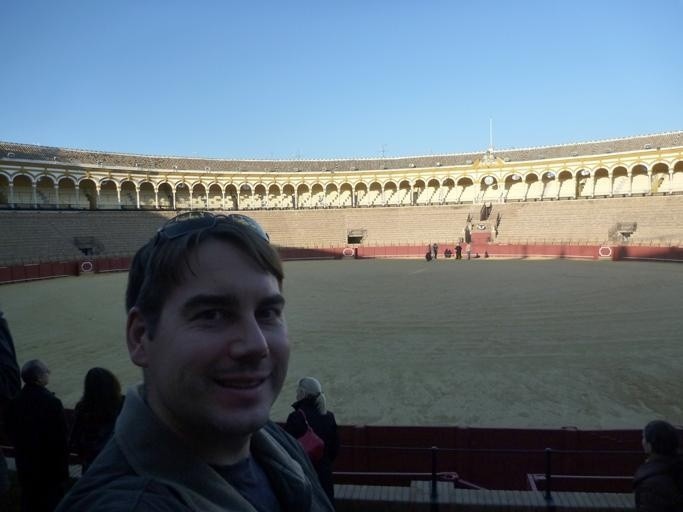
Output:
[71,367,126,476]
[285,376,337,511]
[53,211,334,510]
[425,242,471,261]
[0,310,21,511]
[634,419,682,510]
[13,359,70,511]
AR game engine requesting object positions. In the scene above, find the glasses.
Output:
[155,212,270,243]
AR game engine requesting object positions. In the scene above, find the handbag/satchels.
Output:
[297,428,325,462]
